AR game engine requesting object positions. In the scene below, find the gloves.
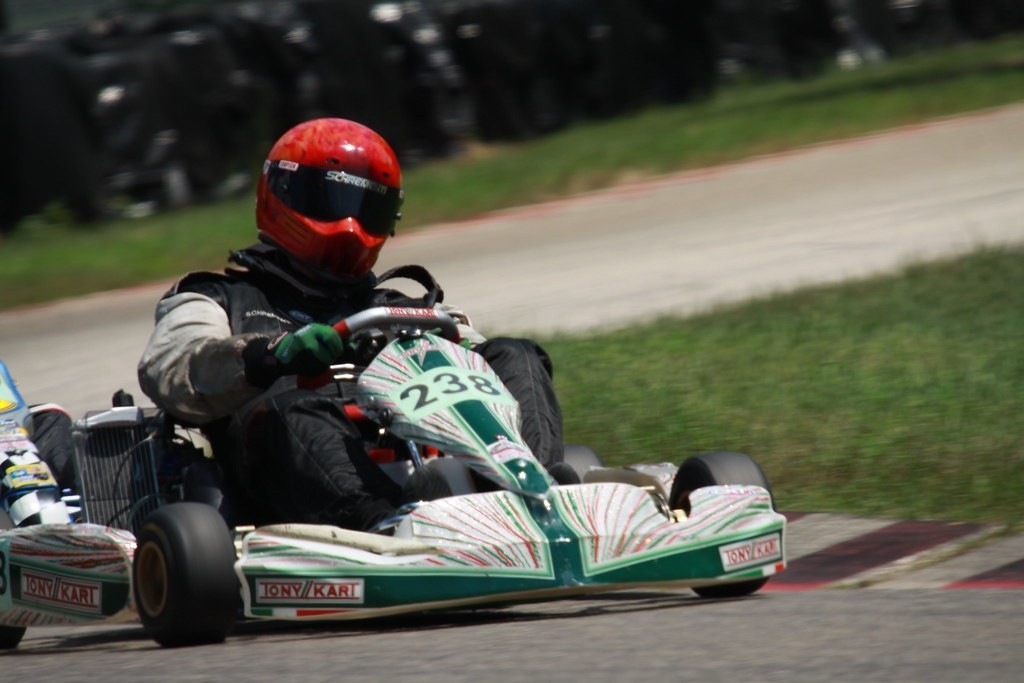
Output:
[245,322,343,390]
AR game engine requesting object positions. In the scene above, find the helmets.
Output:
[255,118,405,284]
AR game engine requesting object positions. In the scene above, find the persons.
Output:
[137,120,582,534]
